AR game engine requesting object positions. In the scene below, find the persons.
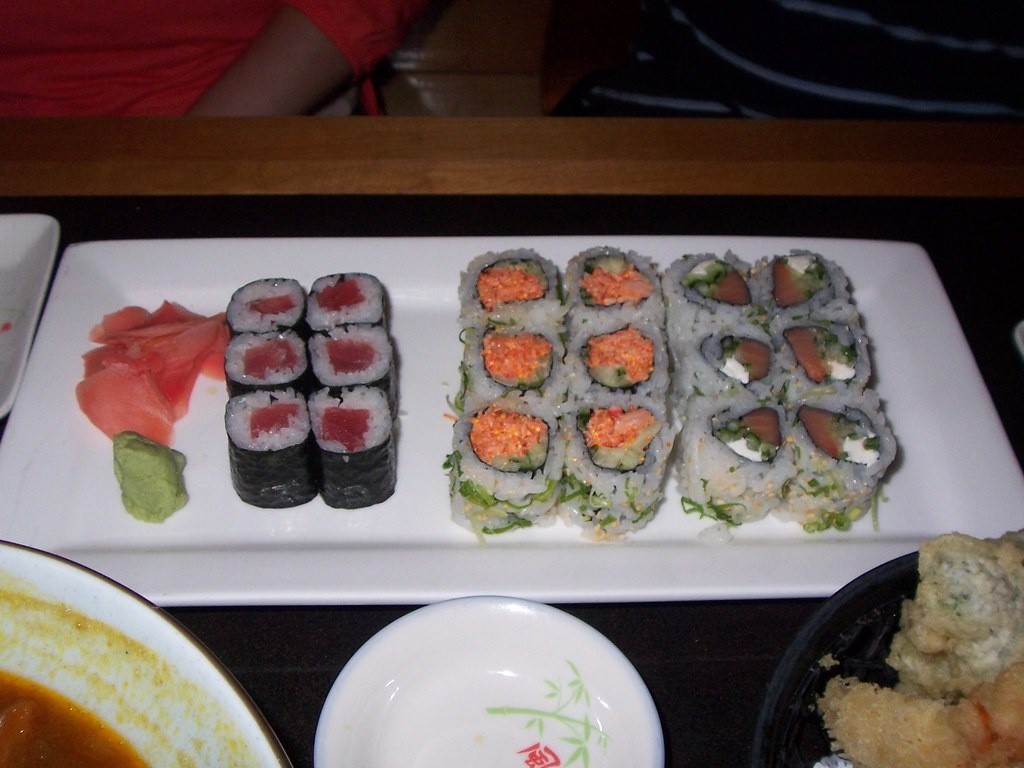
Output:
[541,1,1024,119]
[0,0,427,119]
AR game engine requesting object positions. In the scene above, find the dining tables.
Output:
[0,120,1022,768]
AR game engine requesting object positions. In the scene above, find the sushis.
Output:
[223,245,899,540]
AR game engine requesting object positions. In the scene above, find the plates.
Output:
[752,549,925,766]
[0,232,1023,608]
[310,597,663,768]
[0,213,57,418]
[0,540,294,766]
